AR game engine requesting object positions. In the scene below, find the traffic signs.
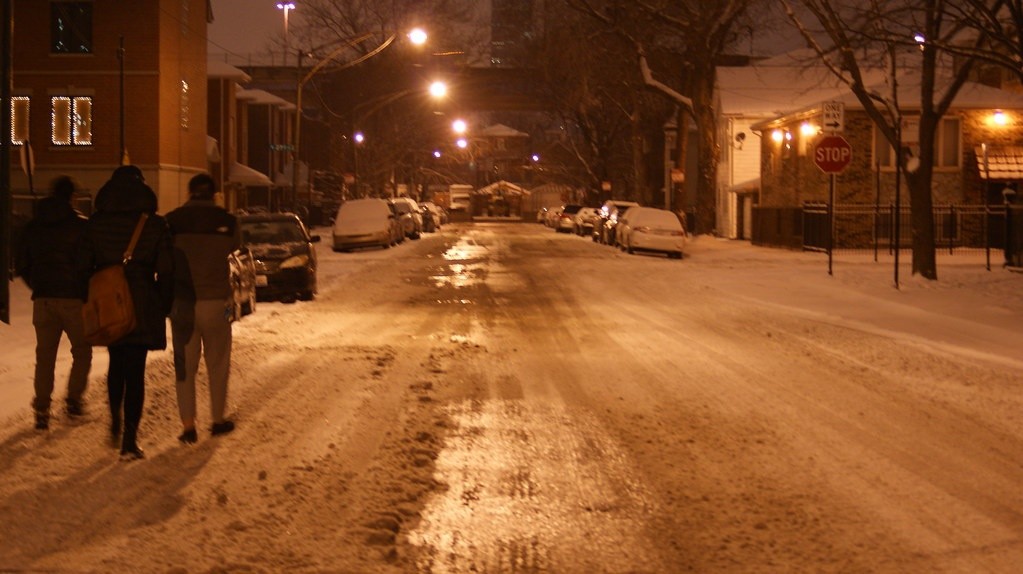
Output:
[821,100,844,132]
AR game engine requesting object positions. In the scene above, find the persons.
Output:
[16,175,93,431]
[83,165,177,460]
[158,173,239,443]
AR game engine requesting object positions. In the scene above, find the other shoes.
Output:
[178,429,197,443]
[120,441,144,460]
[213,421,234,435]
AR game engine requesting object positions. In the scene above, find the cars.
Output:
[603,205,633,245]
[390,198,422,239]
[615,207,640,247]
[238,213,317,299]
[537,204,571,229]
[619,207,685,259]
[227,248,257,319]
[333,197,397,251]
[415,201,447,233]
[557,205,588,233]
[576,208,600,238]
[386,199,406,243]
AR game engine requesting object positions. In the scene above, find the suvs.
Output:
[592,200,637,243]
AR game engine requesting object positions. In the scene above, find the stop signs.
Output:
[815,133,852,173]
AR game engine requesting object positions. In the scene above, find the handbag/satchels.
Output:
[80,265,138,346]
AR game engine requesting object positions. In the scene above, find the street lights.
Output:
[348,76,447,198]
[290,28,428,228]
[278,2,295,64]
[891,29,925,290]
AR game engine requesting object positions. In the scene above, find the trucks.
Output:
[449,184,473,213]
